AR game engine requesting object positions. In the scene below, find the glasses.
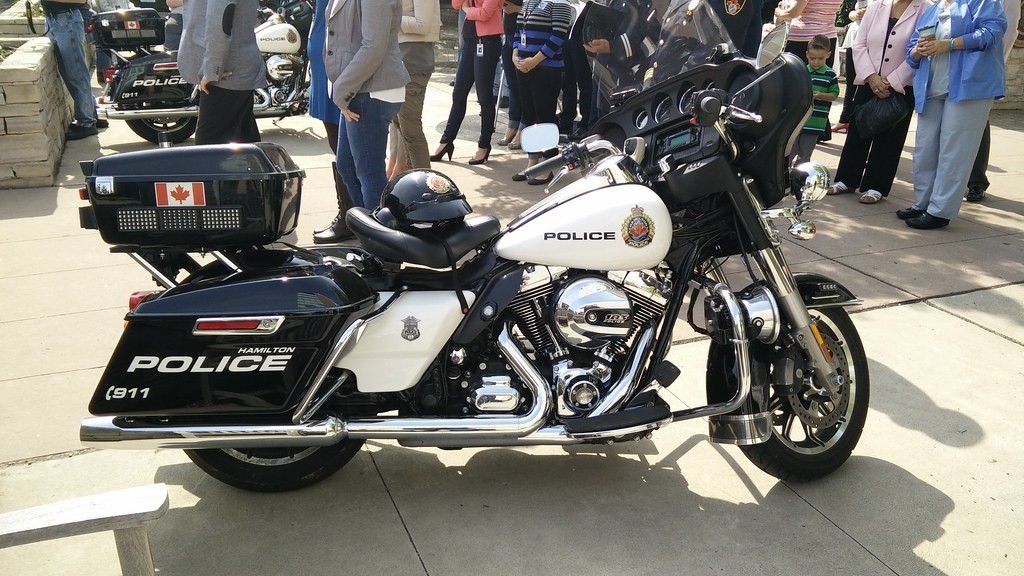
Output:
[368,206,411,234]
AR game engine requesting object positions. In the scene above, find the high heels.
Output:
[428,143,455,162]
[468,143,491,165]
[831,122,849,132]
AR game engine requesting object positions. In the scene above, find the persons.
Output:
[322,0,411,211]
[477,62,510,108]
[762,0,1022,229]
[790,34,840,167]
[512,0,571,185]
[385,0,441,180]
[498,0,526,149]
[558,0,778,140]
[306,0,360,244]
[177,0,267,145]
[40,0,108,140]
[165,0,183,50]
[86,0,130,82]
[429,0,505,165]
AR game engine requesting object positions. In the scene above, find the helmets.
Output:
[386,168,474,232]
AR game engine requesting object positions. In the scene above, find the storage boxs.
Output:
[85,142,305,250]
[92,8,164,49]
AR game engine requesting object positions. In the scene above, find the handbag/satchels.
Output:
[850,84,908,141]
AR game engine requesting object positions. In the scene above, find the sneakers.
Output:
[895,206,948,230]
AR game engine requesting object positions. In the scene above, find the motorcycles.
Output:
[76,38,875,496]
[84,0,333,146]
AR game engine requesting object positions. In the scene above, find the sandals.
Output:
[824,182,853,195]
[859,190,883,203]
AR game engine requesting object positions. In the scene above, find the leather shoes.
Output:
[512,171,553,185]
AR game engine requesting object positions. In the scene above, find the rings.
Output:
[875,89,878,92]
[526,68,528,71]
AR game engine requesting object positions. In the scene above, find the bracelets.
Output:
[950,38,953,50]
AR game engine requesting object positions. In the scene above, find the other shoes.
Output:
[497,129,523,149]
[966,184,987,202]
[65,117,108,140]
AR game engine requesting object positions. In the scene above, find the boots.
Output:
[313,161,361,245]
